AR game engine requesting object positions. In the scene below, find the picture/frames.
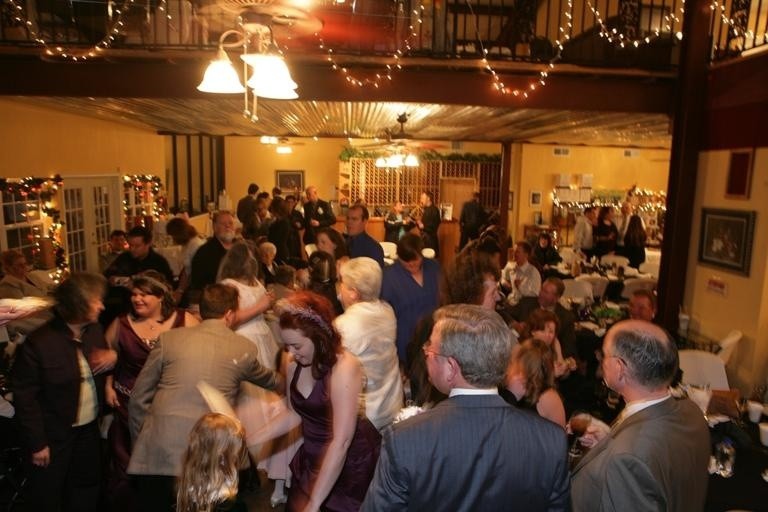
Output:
[507,191,542,226]
[697,206,757,278]
[723,147,756,200]
[275,169,305,194]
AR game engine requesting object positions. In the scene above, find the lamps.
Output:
[377,111,421,174]
[276,136,292,154]
[198,12,298,124]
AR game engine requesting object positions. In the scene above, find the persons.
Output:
[0,181,715,511]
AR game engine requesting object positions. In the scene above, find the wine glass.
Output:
[568,409,592,460]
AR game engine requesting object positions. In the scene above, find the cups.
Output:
[757,421,768,446]
[678,314,689,333]
[748,402,762,425]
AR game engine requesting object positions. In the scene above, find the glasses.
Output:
[596,350,627,367]
[423,341,462,367]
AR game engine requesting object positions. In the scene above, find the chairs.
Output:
[556,247,656,303]
[676,349,731,415]
[715,329,743,366]
